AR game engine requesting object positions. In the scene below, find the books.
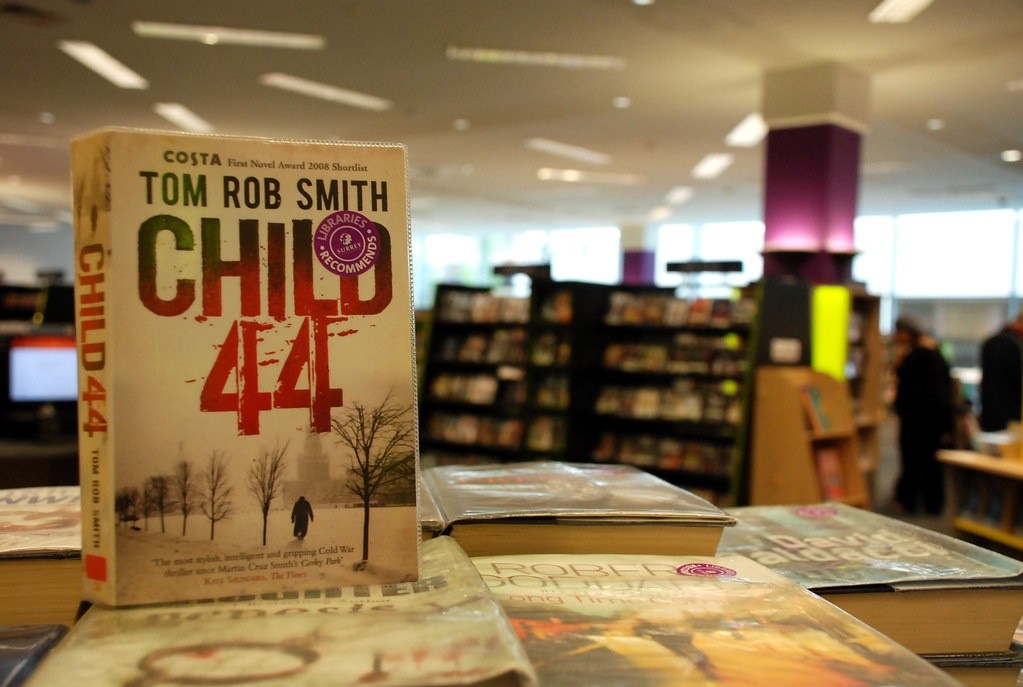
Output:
[1,288,1023,685]
[71,127,421,607]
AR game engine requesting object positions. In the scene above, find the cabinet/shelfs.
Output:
[416,276,872,517]
